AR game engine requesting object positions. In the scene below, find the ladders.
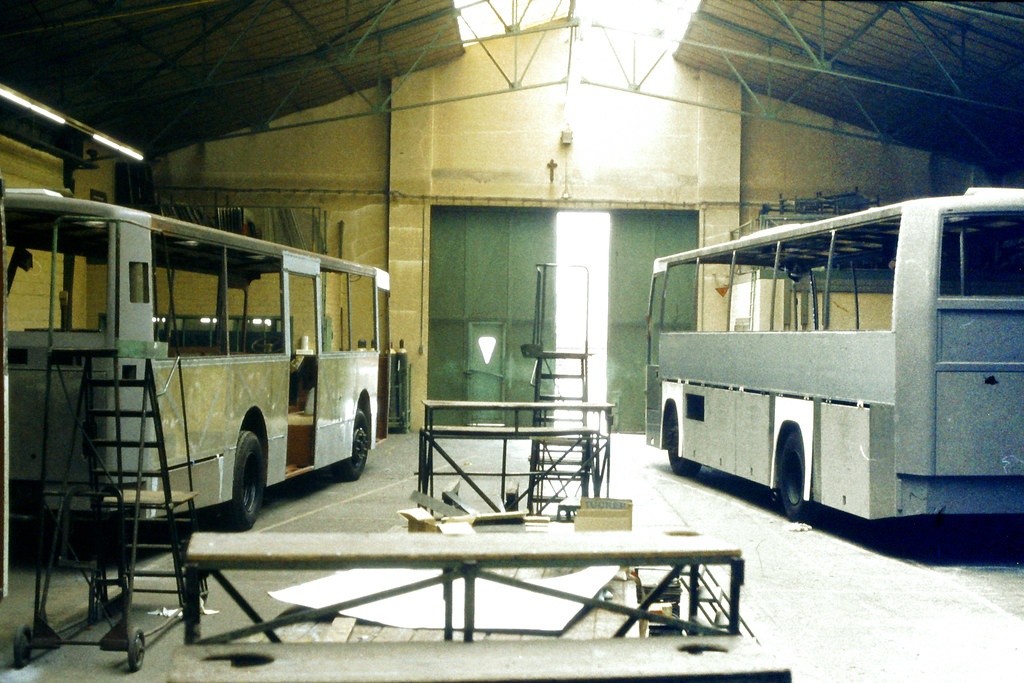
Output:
[12,357,208,672]
[521,263,594,517]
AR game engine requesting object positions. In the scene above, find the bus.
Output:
[643,185,1023,526]
[0,188,398,532]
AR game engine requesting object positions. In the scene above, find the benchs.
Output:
[417,425,600,515]
[162,633,792,683]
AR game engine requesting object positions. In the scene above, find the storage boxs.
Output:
[399,507,438,533]
[574,496,633,530]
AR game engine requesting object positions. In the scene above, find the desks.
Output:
[419,399,616,516]
[183,523,745,645]
